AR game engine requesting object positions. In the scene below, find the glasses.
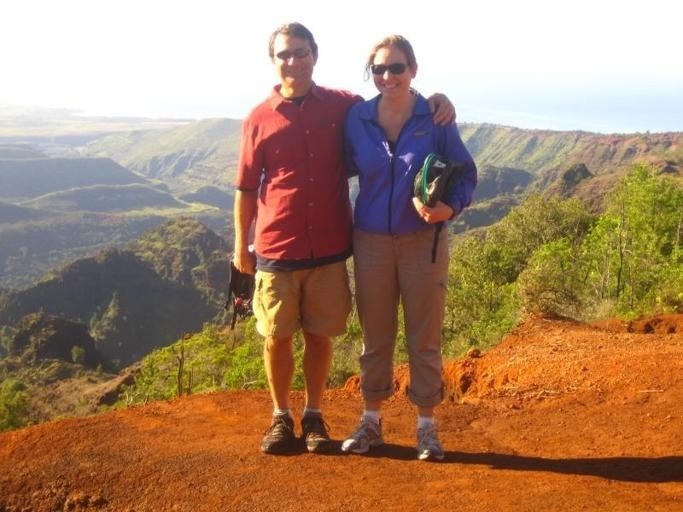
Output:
[276,47,312,60]
[370,63,409,74]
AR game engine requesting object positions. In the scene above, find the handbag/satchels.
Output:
[412,152,474,264]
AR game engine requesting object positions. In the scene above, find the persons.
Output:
[334,32,481,467]
[231,22,458,458]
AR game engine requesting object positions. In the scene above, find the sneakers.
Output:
[261,412,296,454]
[340,412,385,454]
[415,422,445,462]
[300,411,335,453]
